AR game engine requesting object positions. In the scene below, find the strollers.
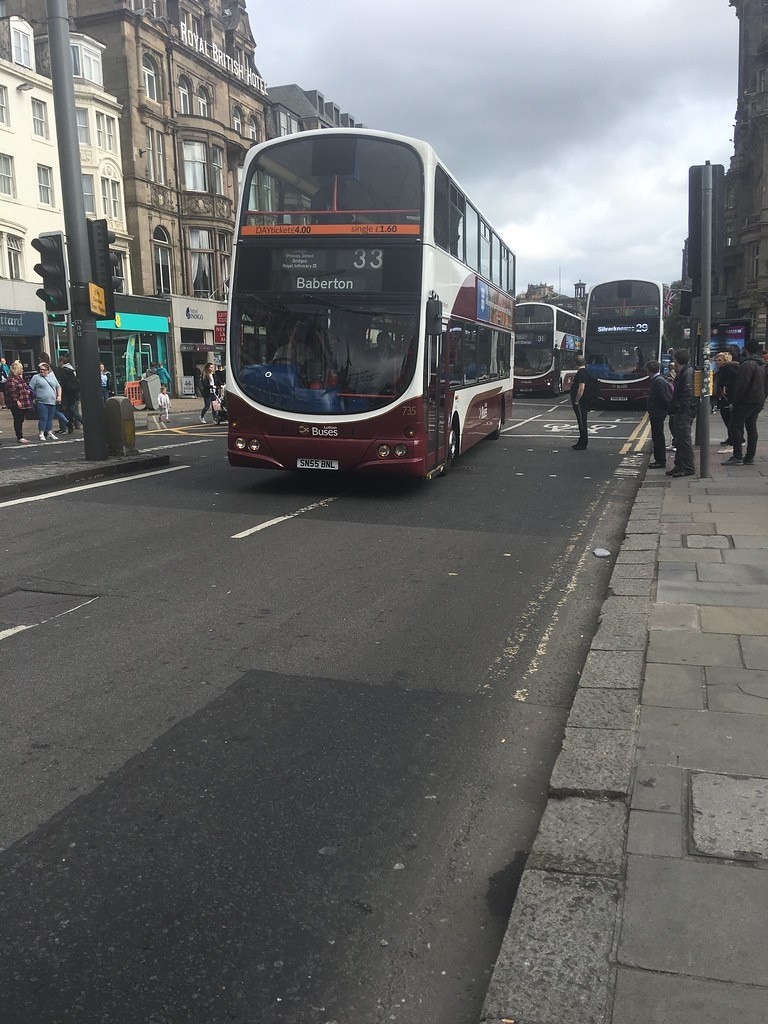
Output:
[210,385,228,426]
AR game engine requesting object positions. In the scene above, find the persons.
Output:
[569,355,591,450]
[664,338,768,478]
[646,360,670,469]
[271,328,314,369]
[0,352,226,443]
[365,333,393,371]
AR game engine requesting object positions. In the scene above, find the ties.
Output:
[291,347,297,362]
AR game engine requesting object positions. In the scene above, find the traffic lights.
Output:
[86,218,121,319]
[31,230,71,316]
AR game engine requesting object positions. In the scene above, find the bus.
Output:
[225,128,516,482]
[513,302,585,397]
[583,280,665,407]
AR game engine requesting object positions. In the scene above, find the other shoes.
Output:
[742,456,755,465]
[718,446,734,454]
[720,439,731,446]
[666,445,677,452]
[572,444,587,451]
[166,419,169,422]
[199,416,206,424]
[19,438,30,444]
[665,467,680,475]
[38,434,46,441]
[78,417,82,424]
[55,429,65,434]
[720,457,743,466]
[742,440,745,447]
[67,421,73,435]
[2,405,6,408]
[648,462,665,468]
[47,432,58,440]
[213,420,218,424]
[672,470,695,477]
[159,418,162,422]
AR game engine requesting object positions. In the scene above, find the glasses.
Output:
[717,359,724,362]
[40,369,44,370]
[288,333,298,337]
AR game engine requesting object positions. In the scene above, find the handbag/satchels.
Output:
[212,398,221,412]
